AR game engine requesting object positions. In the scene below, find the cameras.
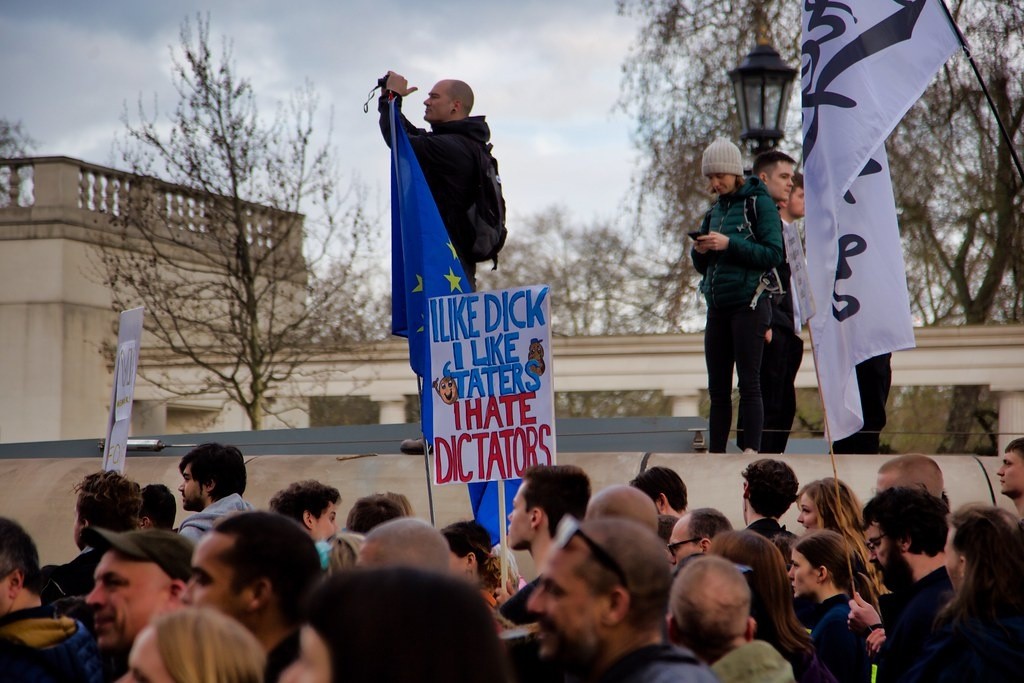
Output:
[377,75,389,88]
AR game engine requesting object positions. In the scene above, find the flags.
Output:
[389,108,523,550]
[799,0,963,439]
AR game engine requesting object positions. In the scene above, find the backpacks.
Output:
[434,133,507,271]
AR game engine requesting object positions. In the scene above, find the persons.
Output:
[379,70,507,455]
[828,352,899,454]
[688,137,809,455]
[0,437,1024,683]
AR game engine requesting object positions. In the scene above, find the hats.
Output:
[702,136,744,176]
[81,525,194,583]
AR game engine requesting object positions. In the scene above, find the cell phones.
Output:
[688,231,704,243]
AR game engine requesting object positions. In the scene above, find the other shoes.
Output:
[400,439,433,455]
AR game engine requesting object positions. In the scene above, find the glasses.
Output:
[556,513,636,610]
[667,537,702,556]
[865,533,885,552]
